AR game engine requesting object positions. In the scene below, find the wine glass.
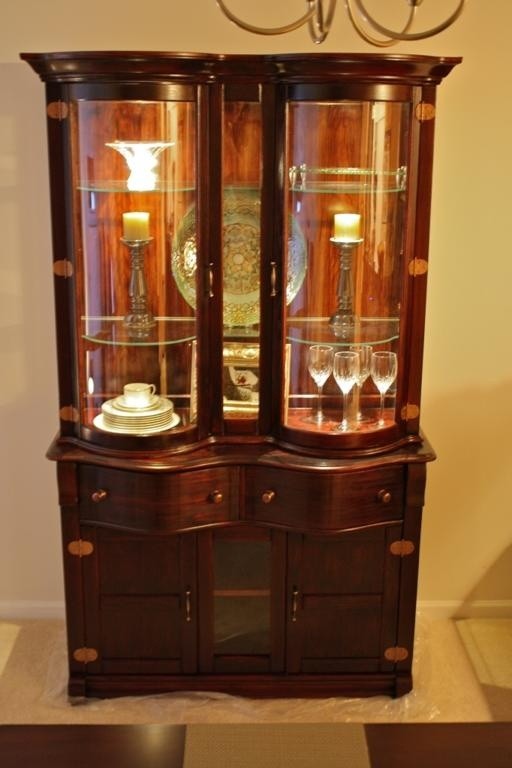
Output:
[299,345,335,426]
[368,351,398,431]
[328,350,362,433]
[349,345,373,425]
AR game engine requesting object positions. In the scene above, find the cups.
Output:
[123,382,156,406]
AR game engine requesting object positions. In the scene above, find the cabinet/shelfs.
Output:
[17,49,465,709]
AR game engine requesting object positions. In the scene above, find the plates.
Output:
[93,394,181,434]
